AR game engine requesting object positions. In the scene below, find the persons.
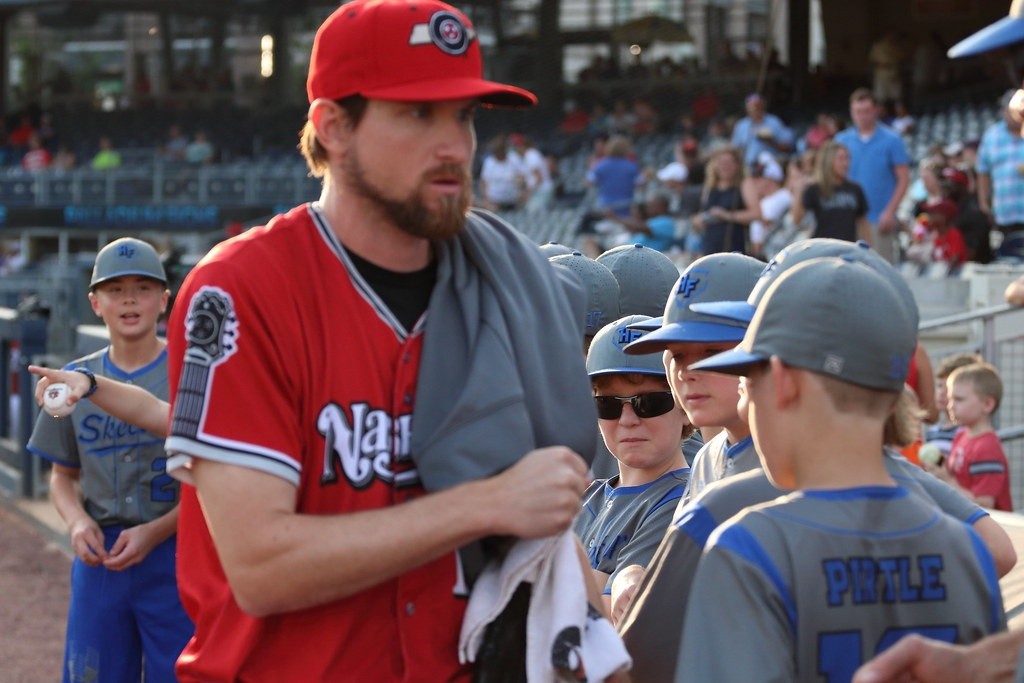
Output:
[477,35,1024,306]
[56,142,77,170]
[159,122,187,157]
[88,133,122,170]
[188,129,217,164]
[29,363,173,442]
[164,0,632,683]
[27,237,197,683]
[521,236,1023,683]
[22,126,52,170]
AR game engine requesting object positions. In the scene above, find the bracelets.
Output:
[72,366,97,399]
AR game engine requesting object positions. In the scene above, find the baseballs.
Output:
[42,383,76,418]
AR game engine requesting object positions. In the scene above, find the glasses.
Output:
[594,390,675,420]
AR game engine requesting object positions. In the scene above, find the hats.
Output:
[686,254,916,393]
[584,314,666,376]
[549,251,623,334]
[90,237,167,286]
[624,251,769,356]
[539,241,583,258]
[627,316,664,332]
[307,0,540,109]
[688,236,919,332]
[593,242,680,320]
